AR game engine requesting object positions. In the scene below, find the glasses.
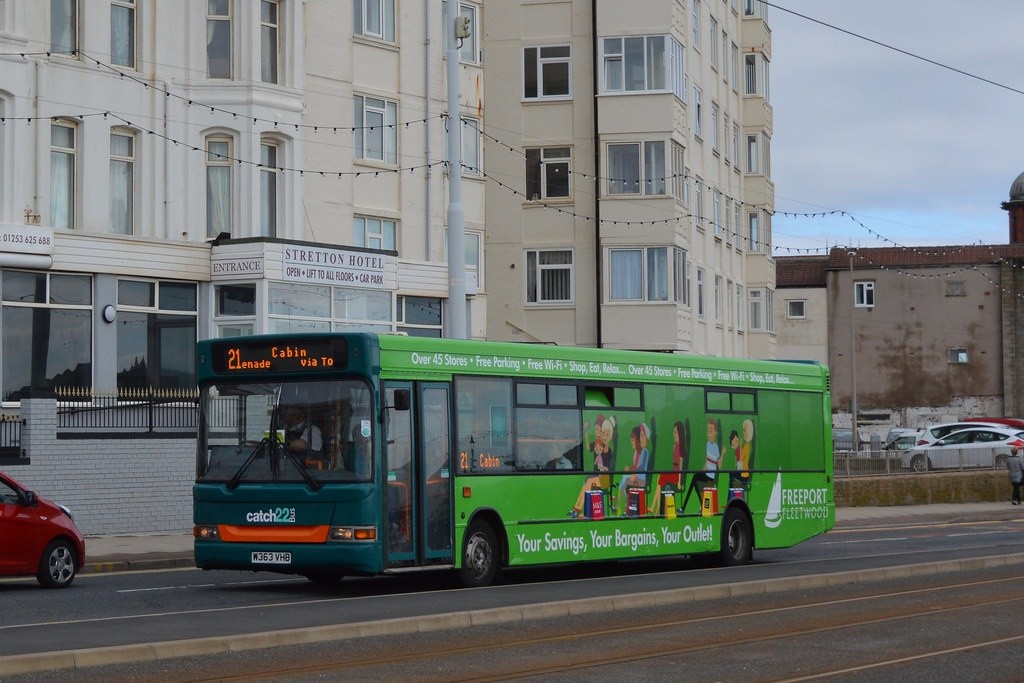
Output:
[286,411,303,416]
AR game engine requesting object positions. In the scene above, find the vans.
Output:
[885,428,917,445]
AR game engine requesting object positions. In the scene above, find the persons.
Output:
[539,440,573,471]
[1007,447,1024,505]
[283,402,324,469]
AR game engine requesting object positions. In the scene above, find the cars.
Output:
[859,432,920,459]
[900,428,1023,470]
[917,422,1021,448]
[0,473,86,590]
[960,418,1024,430]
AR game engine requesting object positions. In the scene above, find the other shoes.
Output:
[1018,500,1022,504]
[1011,500,1017,505]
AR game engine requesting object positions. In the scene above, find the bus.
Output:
[193,333,837,589]
